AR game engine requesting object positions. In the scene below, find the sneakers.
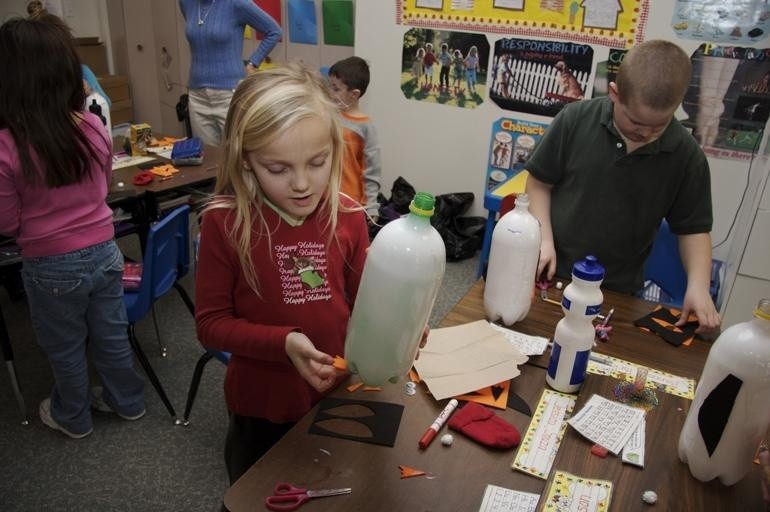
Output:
[88,386,146,420]
[38,398,94,439]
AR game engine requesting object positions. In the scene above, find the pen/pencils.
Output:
[602,308,615,327]
[543,299,605,318]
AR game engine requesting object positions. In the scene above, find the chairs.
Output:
[182,326,233,433]
[75,205,194,421]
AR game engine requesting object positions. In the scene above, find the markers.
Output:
[419,398,458,448]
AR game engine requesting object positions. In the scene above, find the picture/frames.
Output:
[484,117,554,192]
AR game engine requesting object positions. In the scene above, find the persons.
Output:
[196,67,429,488]
[499,143,511,165]
[328,56,381,227]
[464,46,479,92]
[496,55,512,100]
[0,18,147,439]
[524,39,722,335]
[411,48,424,87]
[437,44,453,87]
[494,142,504,165]
[179,1,283,147]
[422,43,439,88]
[451,50,465,88]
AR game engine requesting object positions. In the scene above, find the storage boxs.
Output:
[72,37,109,79]
[631,252,728,308]
[95,75,129,102]
[99,100,137,122]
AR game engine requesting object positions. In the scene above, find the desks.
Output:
[1,124,236,426]
[476,168,536,278]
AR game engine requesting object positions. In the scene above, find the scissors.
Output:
[536,276,555,299]
[266,481,352,512]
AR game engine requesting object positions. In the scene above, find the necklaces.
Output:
[198,0,214,25]
